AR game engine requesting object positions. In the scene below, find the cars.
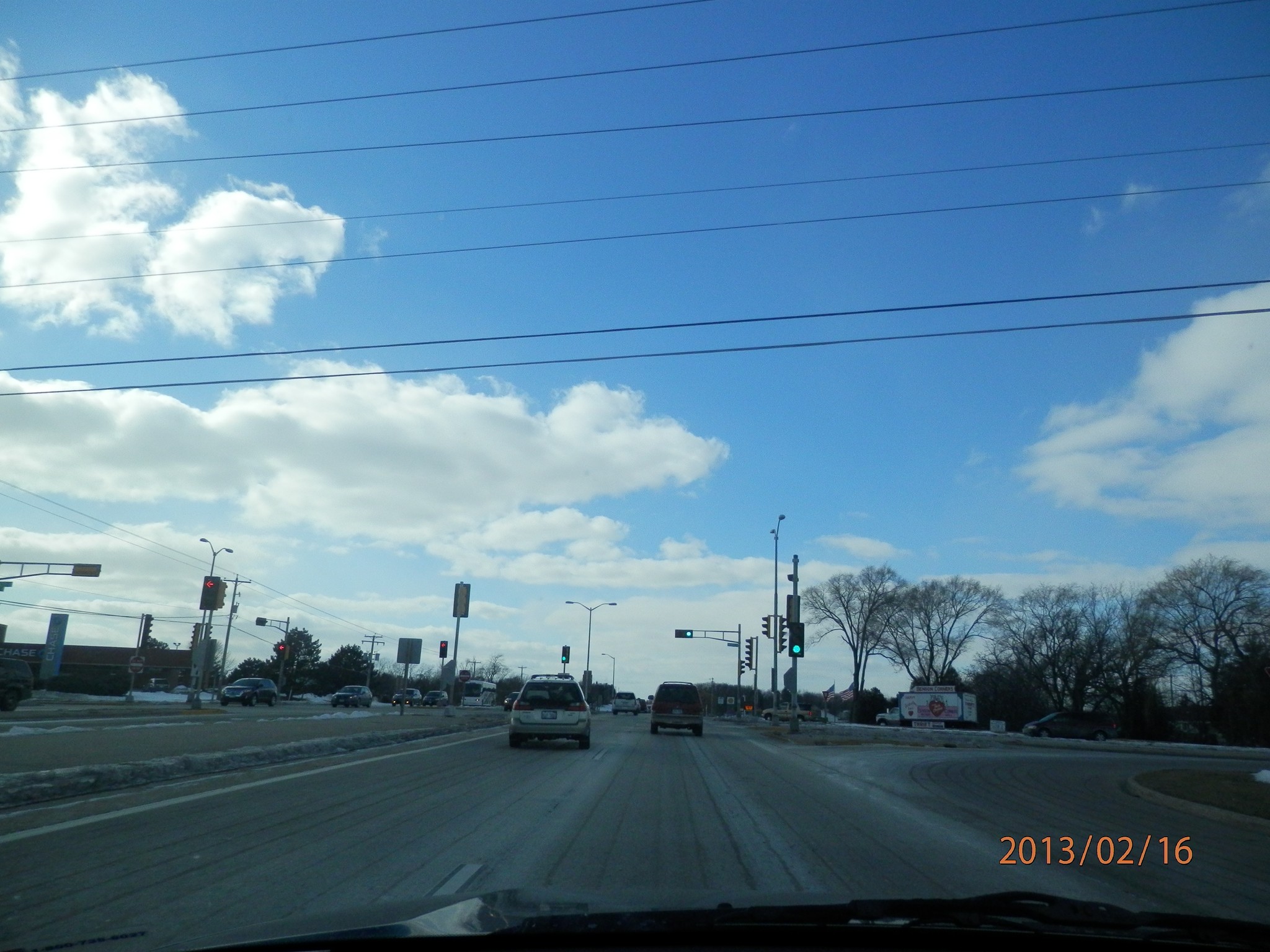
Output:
[0,654,35,712]
[1022,711,1120,742]
[331,686,373,708]
[503,692,520,712]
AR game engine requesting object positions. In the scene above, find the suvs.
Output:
[508,674,591,750]
[761,701,823,722]
[220,678,278,707]
[392,688,423,707]
[143,678,170,693]
[611,690,653,714]
[648,682,704,735]
[422,690,450,707]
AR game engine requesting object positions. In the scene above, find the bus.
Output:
[461,680,497,707]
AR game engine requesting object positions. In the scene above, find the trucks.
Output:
[875,685,978,729]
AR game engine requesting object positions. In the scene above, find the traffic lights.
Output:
[278,643,285,659]
[779,616,805,658]
[740,636,754,676]
[675,630,693,638]
[439,641,448,658]
[561,646,570,663]
[199,576,220,611]
[216,579,228,610]
[761,615,771,638]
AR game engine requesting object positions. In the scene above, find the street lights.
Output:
[173,642,181,650]
[565,601,617,671]
[601,653,615,698]
[770,511,786,709]
[187,538,234,701]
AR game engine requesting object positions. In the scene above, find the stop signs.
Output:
[459,670,471,681]
